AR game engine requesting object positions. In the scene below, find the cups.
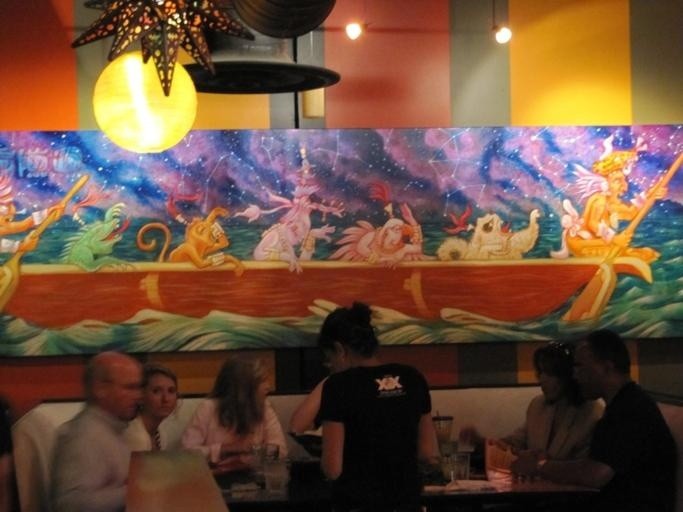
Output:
[434,416,454,447]
[451,452,471,483]
[262,457,292,495]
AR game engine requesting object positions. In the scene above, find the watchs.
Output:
[536,460,548,475]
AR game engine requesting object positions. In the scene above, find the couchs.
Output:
[10,384,544,511]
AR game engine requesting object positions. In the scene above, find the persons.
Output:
[48,350,145,512]
[290,374,331,433]
[176,351,289,474]
[294,301,442,512]
[124,361,182,456]
[516,328,679,512]
[0,174,66,253]
[524,343,607,459]
[0,394,16,512]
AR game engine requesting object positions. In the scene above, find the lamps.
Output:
[70,1,256,97]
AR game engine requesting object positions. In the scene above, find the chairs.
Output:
[125,448,229,511]
